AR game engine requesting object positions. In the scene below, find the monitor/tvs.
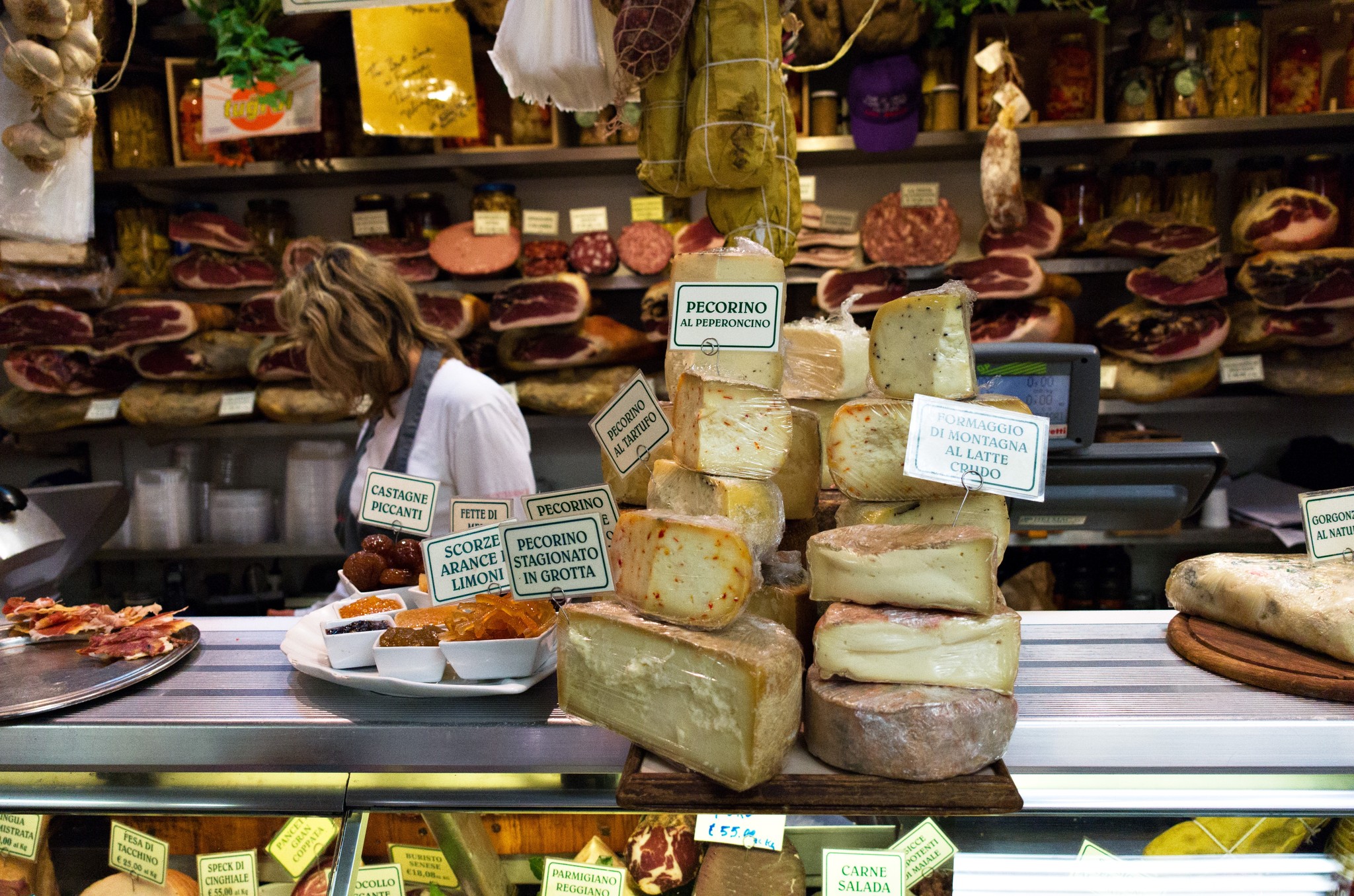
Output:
[997,431,1224,533]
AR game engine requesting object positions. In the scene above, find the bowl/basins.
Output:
[103,440,352,545]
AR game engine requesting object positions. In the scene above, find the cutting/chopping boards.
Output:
[1166,612,1353,704]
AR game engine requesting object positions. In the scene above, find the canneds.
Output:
[240,196,295,256]
[810,89,839,136]
[1015,152,1345,247]
[348,181,523,235]
[110,75,167,168]
[978,5,1354,124]
[932,82,960,131]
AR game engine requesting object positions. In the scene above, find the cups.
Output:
[321,568,564,683]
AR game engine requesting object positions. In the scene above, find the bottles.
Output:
[1199,486,1231,526]
[1240,154,1341,209]
[1021,155,1216,235]
[811,89,838,135]
[932,84,959,132]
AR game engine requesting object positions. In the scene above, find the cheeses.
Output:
[1165,550,1352,664]
[559,284,1021,779]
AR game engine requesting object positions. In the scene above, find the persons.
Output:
[273,235,535,555]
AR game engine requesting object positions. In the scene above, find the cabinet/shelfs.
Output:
[0,0,1354,614]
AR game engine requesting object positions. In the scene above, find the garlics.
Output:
[0,0,105,171]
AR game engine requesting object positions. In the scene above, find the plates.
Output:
[279,599,557,697]
[0,620,201,720]
